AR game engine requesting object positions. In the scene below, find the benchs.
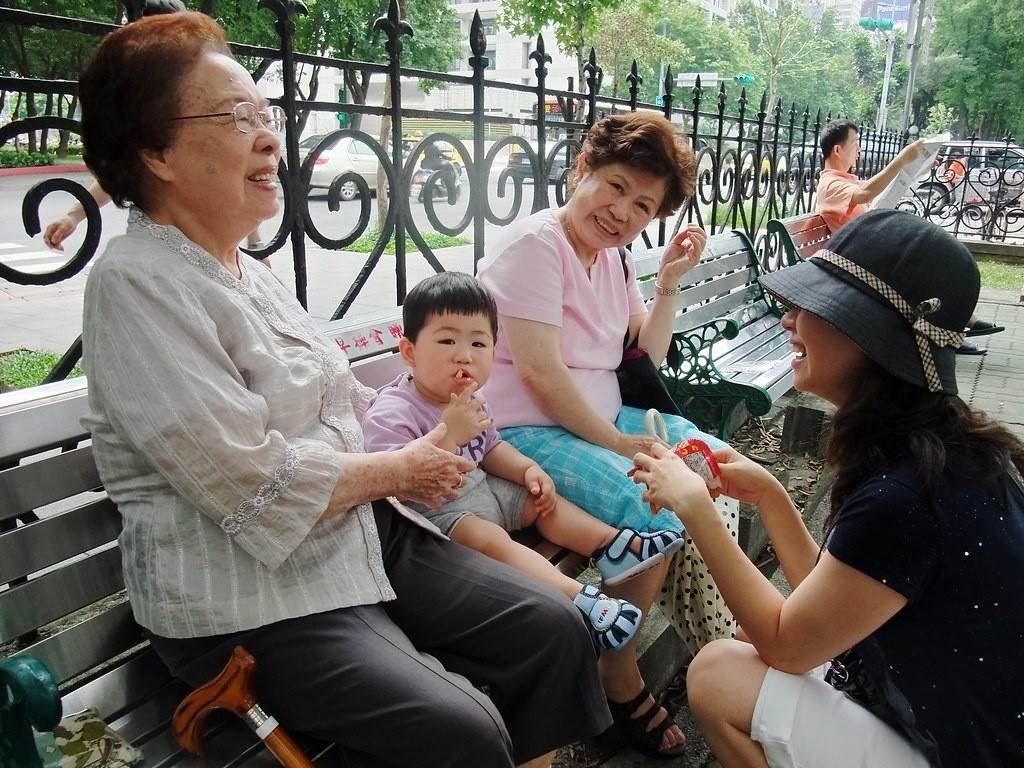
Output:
[769,212,837,264]
[621,225,812,441]
[2,307,628,768]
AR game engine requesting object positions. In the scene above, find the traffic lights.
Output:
[733,75,751,83]
[337,87,351,124]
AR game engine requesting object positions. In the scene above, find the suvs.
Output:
[911,138,1024,217]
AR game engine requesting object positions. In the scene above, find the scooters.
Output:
[412,147,463,204]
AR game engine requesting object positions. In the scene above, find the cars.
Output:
[506,138,570,185]
[400,136,465,178]
[790,134,827,175]
[277,132,421,200]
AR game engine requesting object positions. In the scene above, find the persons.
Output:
[946,152,971,207]
[811,119,1006,358]
[629,211,1020,767]
[361,271,681,652]
[420,139,453,173]
[45,180,273,269]
[479,109,766,754]
[75,10,610,768]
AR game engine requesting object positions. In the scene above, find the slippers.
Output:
[964,320,1005,337]
[954,339,988,355]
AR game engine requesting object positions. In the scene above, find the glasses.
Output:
[160,102,288,134]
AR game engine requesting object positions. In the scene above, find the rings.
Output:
[453,475,464,490]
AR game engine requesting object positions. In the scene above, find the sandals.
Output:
[572,584,643,651]
[589,527,684,587]
[604,685,686,758]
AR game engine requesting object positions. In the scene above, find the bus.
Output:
[529,100,665,145]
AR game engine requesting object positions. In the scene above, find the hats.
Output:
[756,209,980,395]
